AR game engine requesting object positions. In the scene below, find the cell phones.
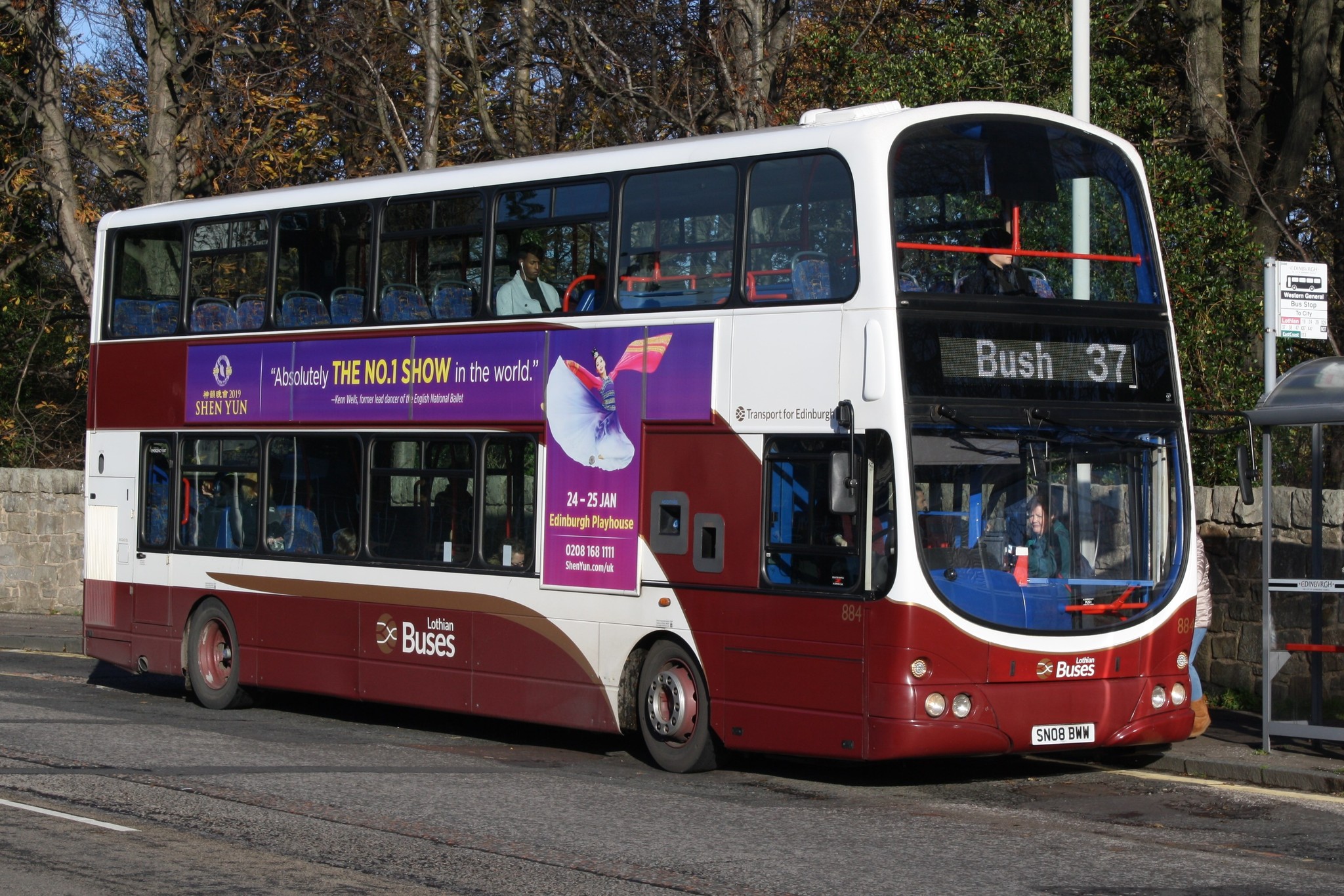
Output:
[553,307,563,312]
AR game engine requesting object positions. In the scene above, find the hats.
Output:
[974,229,1014,261]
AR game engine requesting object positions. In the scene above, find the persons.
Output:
[336,527,357,557]
[496,242,562,316]
[1147,499,1214,737]
[890,483,969,548]
[434,463,473,530]
[541,332,673,472]
[486,537,525,567]
[202,472,285,551]
[960,228,1038,295]
[1024,490,1071,598]
[831,528,847,547]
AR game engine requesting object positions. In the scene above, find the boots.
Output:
[1188,694,1212,738]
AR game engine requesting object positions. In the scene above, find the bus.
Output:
[77,101,1198,773]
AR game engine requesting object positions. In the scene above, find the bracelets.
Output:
[833,533,842,538]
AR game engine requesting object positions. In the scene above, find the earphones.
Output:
[521,262,523,268]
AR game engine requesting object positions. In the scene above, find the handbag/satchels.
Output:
[1080,553,1096,580]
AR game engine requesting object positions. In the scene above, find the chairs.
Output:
[147,466,478,565]
[116,253,1050,334]
